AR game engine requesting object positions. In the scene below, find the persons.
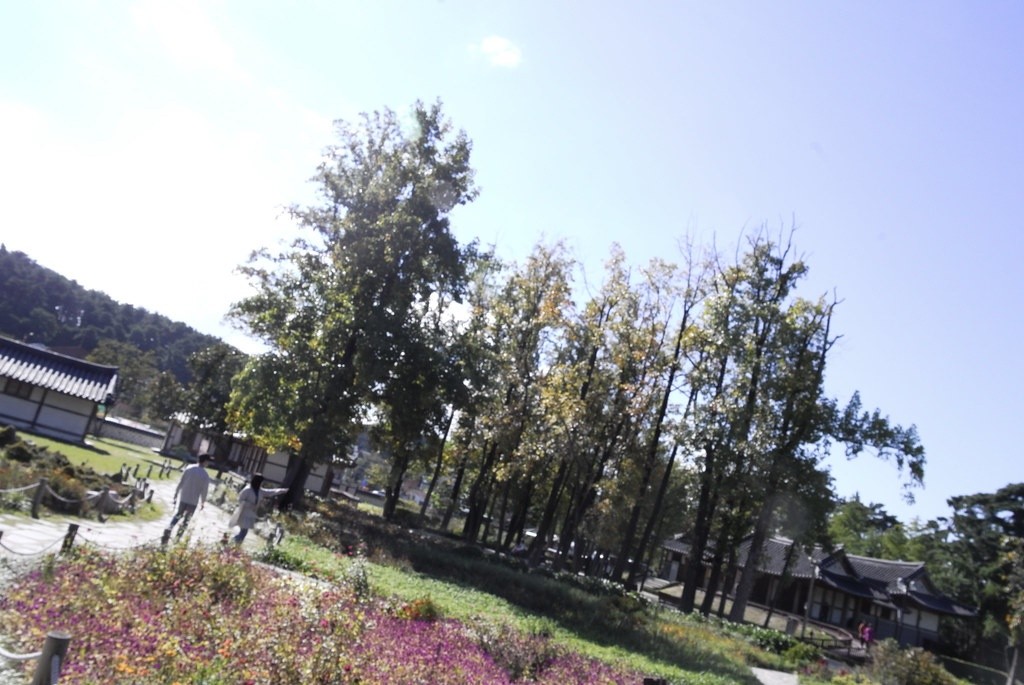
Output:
[227,474,289,545]
[858,619,875,648]
[161,453,209,545]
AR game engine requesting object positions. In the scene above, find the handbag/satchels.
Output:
[228,501,247,528]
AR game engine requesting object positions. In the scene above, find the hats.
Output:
[199,453,216,461]
